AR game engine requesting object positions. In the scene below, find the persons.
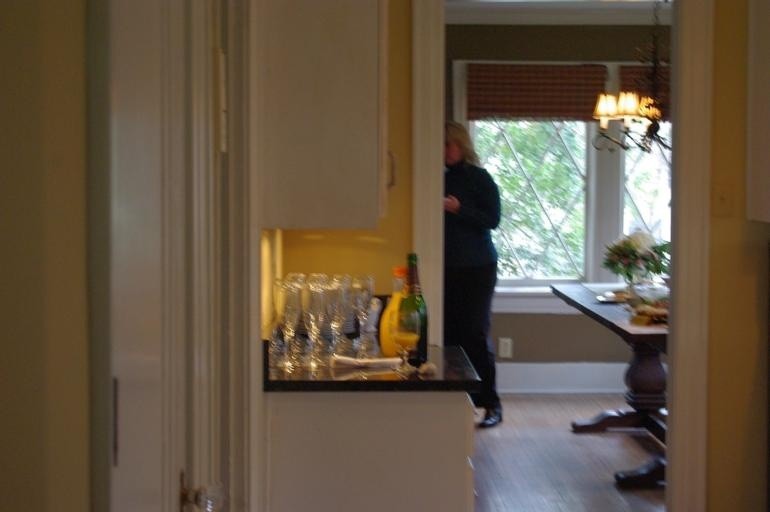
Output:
[441,118,507,429]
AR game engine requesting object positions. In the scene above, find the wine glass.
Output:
[389,309,422,376]
[272,271,383,374]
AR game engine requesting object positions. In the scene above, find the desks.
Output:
[264,343,481,512]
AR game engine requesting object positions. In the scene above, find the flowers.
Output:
[599,229,670,283]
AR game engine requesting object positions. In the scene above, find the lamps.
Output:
[582,1,673,156]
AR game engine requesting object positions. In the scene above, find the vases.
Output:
[623,276,640,301]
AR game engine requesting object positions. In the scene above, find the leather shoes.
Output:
[478,408,503,427]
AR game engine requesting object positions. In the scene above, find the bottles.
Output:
[377,254,428,369]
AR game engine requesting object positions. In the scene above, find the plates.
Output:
[596,295,627,304]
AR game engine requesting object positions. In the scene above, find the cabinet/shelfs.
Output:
[249,0,397,231]
